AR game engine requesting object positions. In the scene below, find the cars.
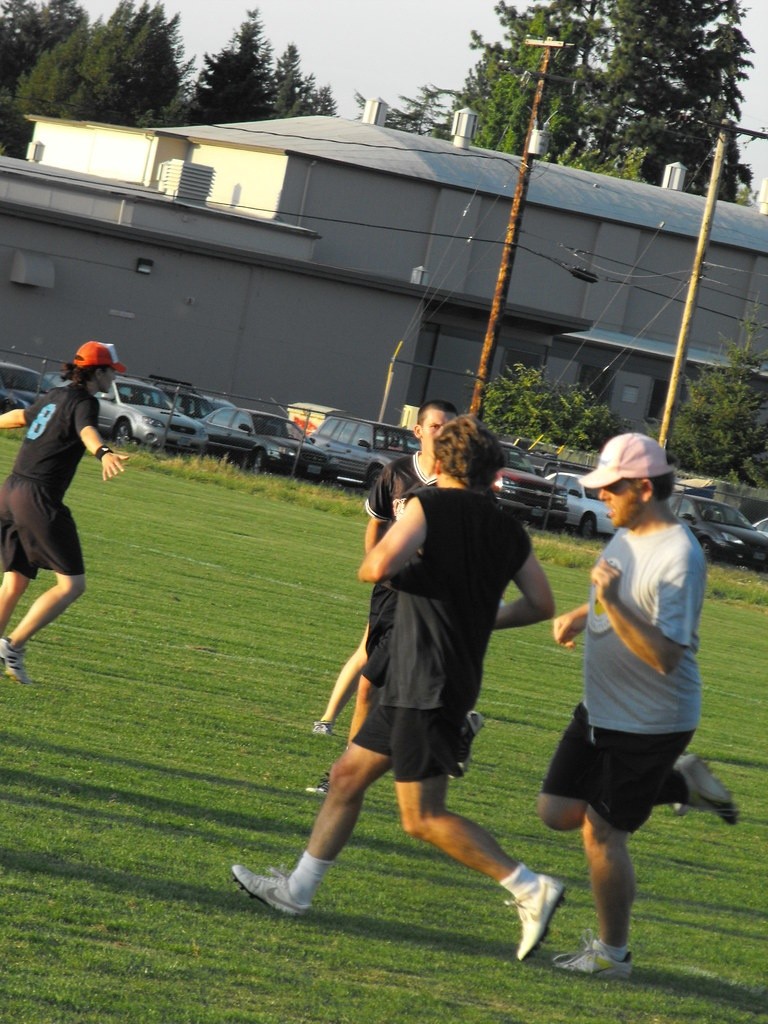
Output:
[0,360,331,484]
[542,472,624,537]
[665,492,767,571]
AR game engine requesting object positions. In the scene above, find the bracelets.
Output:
[95,444,113,461]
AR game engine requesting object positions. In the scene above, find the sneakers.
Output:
[504,874,566,962]
[231,864,313,917]
[551,928,632,981]
[0,636,30,686]
[677,755,738,825]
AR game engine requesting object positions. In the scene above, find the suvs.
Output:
[487,442,596,533]
[305,411,421,490]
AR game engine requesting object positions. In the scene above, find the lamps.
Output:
[136,257,154,274]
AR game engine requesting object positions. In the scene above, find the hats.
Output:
[73,340,127,373]
[576,432,672,488]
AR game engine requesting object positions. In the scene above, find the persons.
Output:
[304,396,487,797]
[541,433,739,977]
[228,412,568,962]
[0,341,130,684]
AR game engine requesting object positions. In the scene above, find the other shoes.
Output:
[305,774,330,793]
[313,720,331,734]
[454,711,483,771]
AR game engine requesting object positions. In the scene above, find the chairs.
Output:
[701,509,721,521]
